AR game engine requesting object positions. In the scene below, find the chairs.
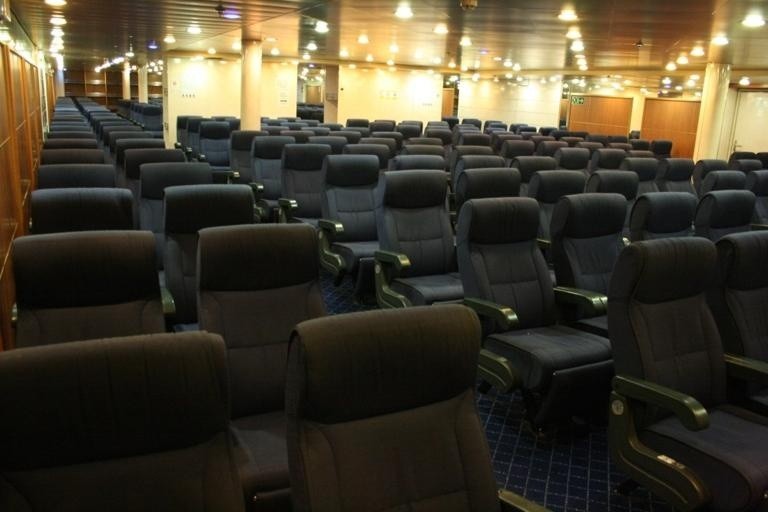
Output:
[0,95,768,511]
